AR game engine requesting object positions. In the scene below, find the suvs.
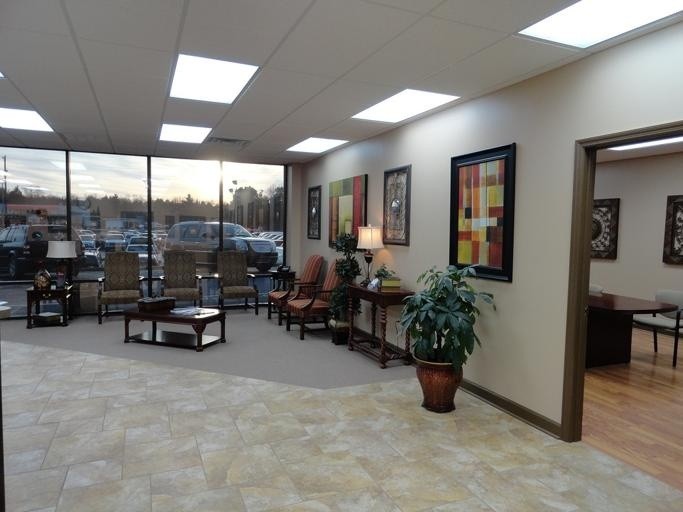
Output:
[0,223,82,279]
[163,220,277,271]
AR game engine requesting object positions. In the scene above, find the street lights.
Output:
[228,179,239,223]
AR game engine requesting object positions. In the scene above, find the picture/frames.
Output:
[381,163,410,246]
[447,142,516,283]
[659,193,682,265]
[588,196,619,260]
[306,185,320,240]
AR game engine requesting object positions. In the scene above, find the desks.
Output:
[585,286,677,369]
[268,269,295,310]
[345,281,414,370]
[25,284,74,329]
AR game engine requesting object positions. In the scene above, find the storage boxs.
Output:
[31,311,63,326]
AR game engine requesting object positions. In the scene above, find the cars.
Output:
[248,228,285,264]
[77,225,168,268]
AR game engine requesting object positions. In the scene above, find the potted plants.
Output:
[372,265,397,292]
[398,263,498,415]
[328,232,362,345]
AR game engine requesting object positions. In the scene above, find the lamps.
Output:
[46,240,78,288]
[356,224,384,287]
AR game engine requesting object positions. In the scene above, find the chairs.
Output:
[630,290,682,367]
[267,255,322,320]
[159,250,202,307]
[286,258,347,340]
[97,251,145,324]
[216,250,258,315]
